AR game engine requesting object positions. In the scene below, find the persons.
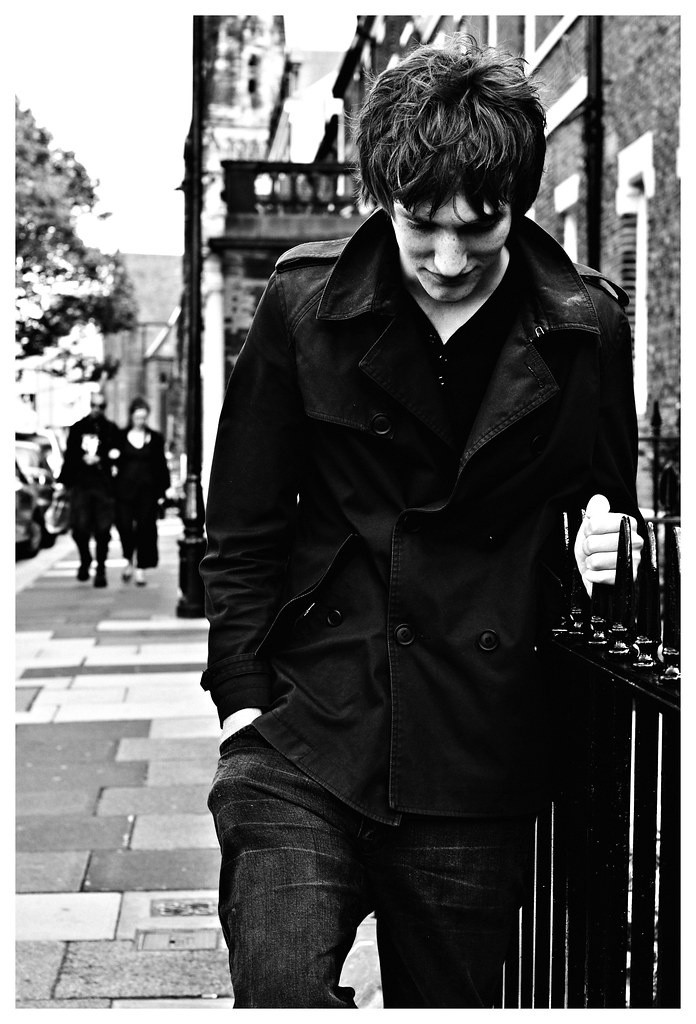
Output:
[201,31,651,1009]
[54,390,171,588]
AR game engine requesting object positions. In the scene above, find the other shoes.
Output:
[77,560,91,581]
[134,568,148,585]
[122,563,135,580]
[94,566,107,588]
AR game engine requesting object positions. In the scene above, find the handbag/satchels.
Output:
[44,482,71,535]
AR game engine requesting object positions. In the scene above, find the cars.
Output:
[16,412,76,562]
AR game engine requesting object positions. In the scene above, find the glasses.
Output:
[91,403,107,410]
